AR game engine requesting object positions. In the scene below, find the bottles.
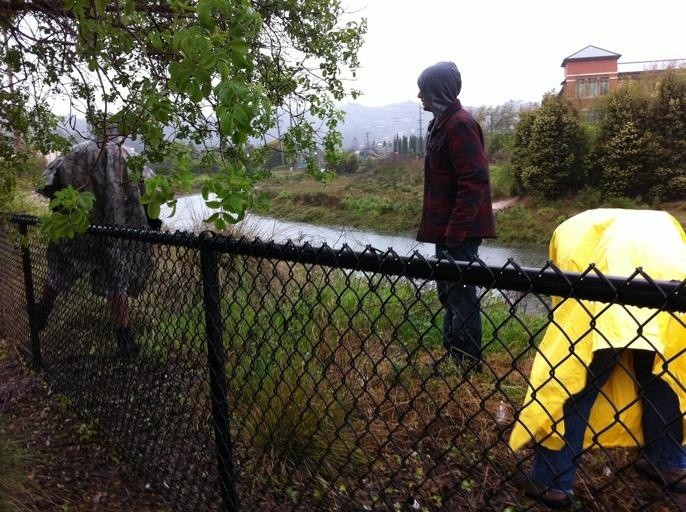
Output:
[497,401,504,417]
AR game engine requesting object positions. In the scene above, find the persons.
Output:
[508,207,686,509]
[417,62,497,379]
[34,112,154,357]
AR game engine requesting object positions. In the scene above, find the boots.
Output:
[35,284,57,328]
[117,323,138,356]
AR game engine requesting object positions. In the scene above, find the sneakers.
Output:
[635,458,686,493]
[516,475,572,508]
